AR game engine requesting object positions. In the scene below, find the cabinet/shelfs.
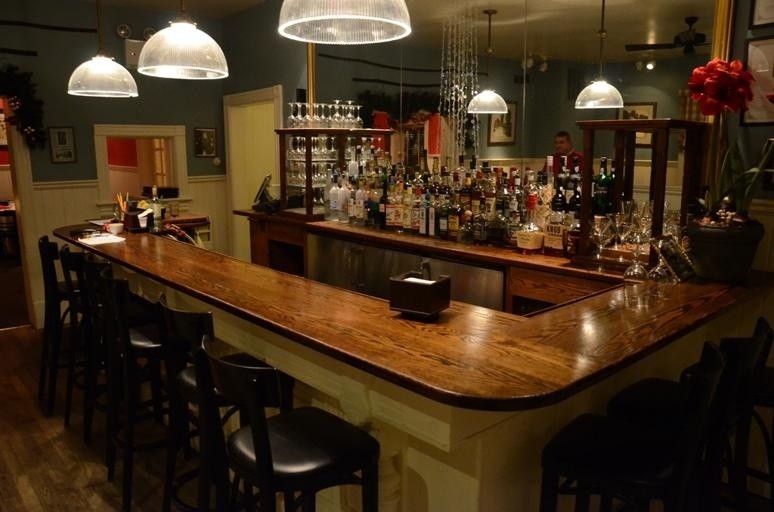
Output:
[575,117,708,274]
[272,129,394,220]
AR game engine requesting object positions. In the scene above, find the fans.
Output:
[625,16,712,53]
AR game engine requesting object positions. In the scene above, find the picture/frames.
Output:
[47,126,77,164]
[614,102,657,148]
[192,127,218,158]
[740,0,774,127]
[487,100,518,147]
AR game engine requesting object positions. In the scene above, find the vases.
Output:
[686,220,764,281]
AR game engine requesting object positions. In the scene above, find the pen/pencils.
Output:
[115,192,129,209]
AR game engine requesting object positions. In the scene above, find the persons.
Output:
[542,131,594,175]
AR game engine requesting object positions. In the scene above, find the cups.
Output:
[169,202,182,218]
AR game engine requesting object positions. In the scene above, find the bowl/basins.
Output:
[108,223,124,234]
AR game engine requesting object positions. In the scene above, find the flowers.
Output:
[687,56,774,217]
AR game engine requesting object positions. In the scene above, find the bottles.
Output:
[321,149,554,245]
[552,177,568,212]
[568,178,582,213]
[150,185,162,220]
[555,155,570,187]
[681,223,693,263]
[609,159,616,210]
[595,157,608,213]
[567,156,583,186]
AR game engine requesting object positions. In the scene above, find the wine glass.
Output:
[618,197,681,224]
[286,137,337,159]
[298,188,323,207]
[287,99,365,129]
[592,216,680,282]
[289,163,326,186]
[345,137,384,159]
